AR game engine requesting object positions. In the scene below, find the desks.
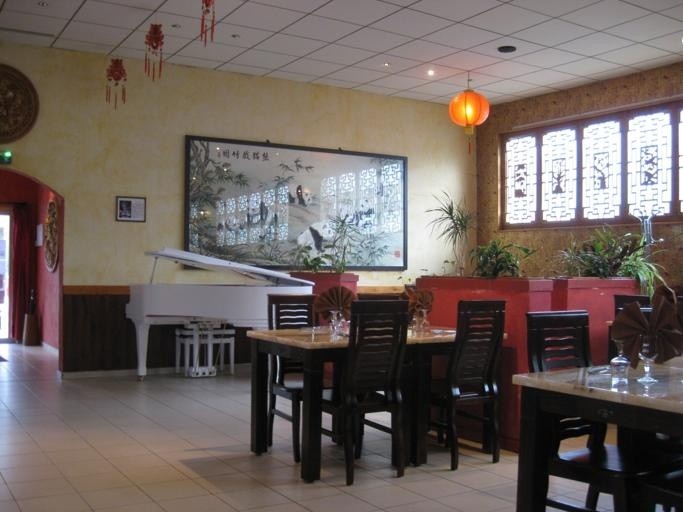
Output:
[248,326,508,485]
[509,360,681,511]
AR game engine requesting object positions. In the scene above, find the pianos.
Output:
[127,247,316,383]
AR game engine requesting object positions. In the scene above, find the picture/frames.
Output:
[114,195,147,223]
[182,131,409,272]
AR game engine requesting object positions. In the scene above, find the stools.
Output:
[635,464,682,512]
[175,327,237,376]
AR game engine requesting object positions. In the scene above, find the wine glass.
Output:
[610,337,630,389]
[408,307,431,336]
[308,309,350,338]
[635,333,659,384]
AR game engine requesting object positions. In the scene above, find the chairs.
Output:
[425,299,507,473]
[525,306,638,511]
[263,291,338,463]
[606,290,683,361]
[317,298,413,488]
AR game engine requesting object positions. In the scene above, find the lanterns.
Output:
[448,90,491,154]
